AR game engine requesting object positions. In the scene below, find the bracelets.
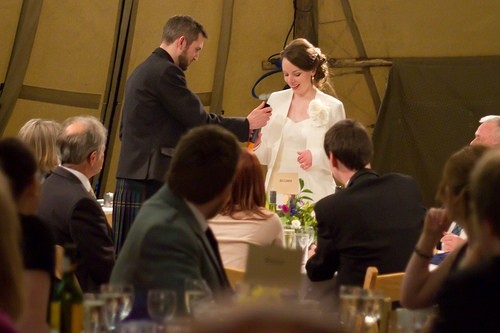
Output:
[414,248,433,259]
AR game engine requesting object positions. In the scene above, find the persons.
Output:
[0,138,54,333]
[112,16,271,249]
[249,37,345,217]
[398,116,500,333]
[306,119,425,292]
[207,149,284,272]
[16,116,114,290]
[109,124,239,333]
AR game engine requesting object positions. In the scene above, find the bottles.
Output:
[51,243,86,333]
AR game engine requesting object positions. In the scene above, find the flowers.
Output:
[278,179,318,242]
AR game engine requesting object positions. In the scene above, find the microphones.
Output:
[247,103,270,151]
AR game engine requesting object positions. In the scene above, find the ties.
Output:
[206,227,222,264]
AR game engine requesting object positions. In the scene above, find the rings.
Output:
[302,163,305,166]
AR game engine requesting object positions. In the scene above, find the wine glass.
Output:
[147,289,178,333]
[359,290,384,333]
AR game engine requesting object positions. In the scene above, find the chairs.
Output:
[364,267,405,302]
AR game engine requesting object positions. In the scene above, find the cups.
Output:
[284,227,314,265]
[84,284,135,333]
